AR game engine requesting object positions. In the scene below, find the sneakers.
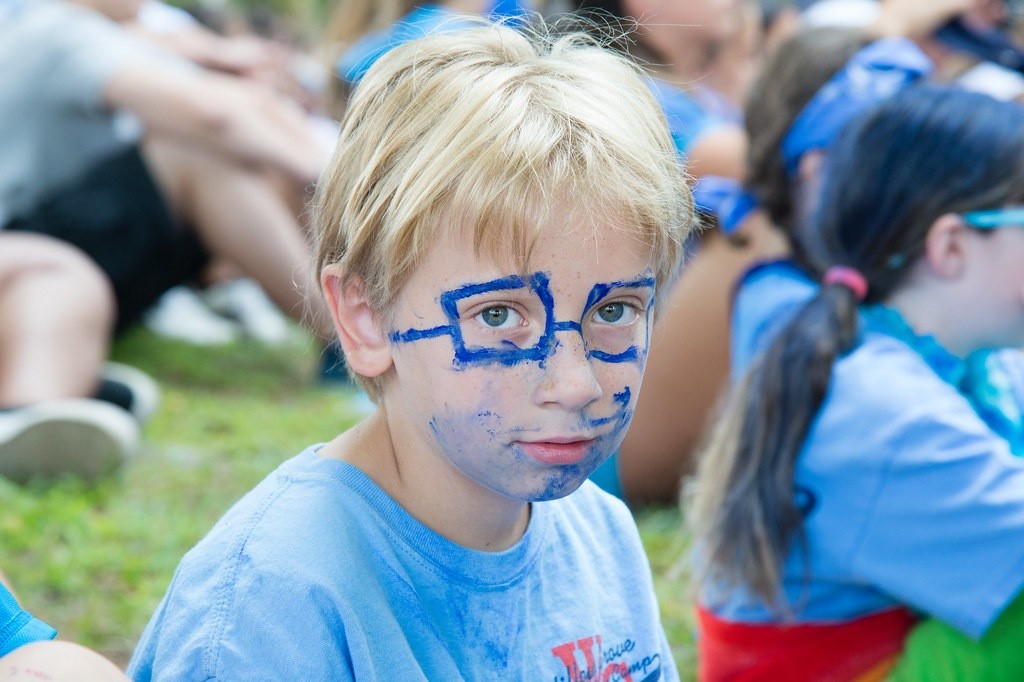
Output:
[147,285,237,347]
[208,277,290,343]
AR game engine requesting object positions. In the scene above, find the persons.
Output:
[1,0,1024,499]
[1,582,135,681]
[126,11,717,682]
[689,85,1023,682]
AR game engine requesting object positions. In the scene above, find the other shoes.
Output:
[96,359,155,421]
[1,397,137,487]
